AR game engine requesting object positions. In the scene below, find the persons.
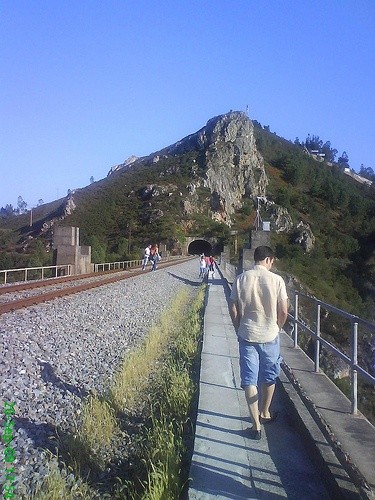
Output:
[197,252,216,279]
[228,246,287,440]
[140,243,162,272]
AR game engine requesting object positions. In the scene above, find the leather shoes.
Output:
[247,426,261,440]
[259,411,278,424]
